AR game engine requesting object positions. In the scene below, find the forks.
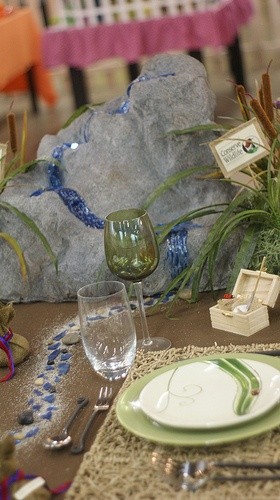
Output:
[70,387,112,454]
[151,452,280,489]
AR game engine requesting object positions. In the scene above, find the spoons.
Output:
[42,397,89,449]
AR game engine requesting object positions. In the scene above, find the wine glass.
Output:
[103,208,172,354]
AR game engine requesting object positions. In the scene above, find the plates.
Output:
[115,353,280,446]
[139,358,280,429]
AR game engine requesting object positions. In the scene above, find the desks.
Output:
[0,293,280,500]
[0,0,253,115]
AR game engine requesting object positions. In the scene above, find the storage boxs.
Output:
[210,269,280,337]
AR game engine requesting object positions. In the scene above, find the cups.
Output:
[77,281,137,382]
